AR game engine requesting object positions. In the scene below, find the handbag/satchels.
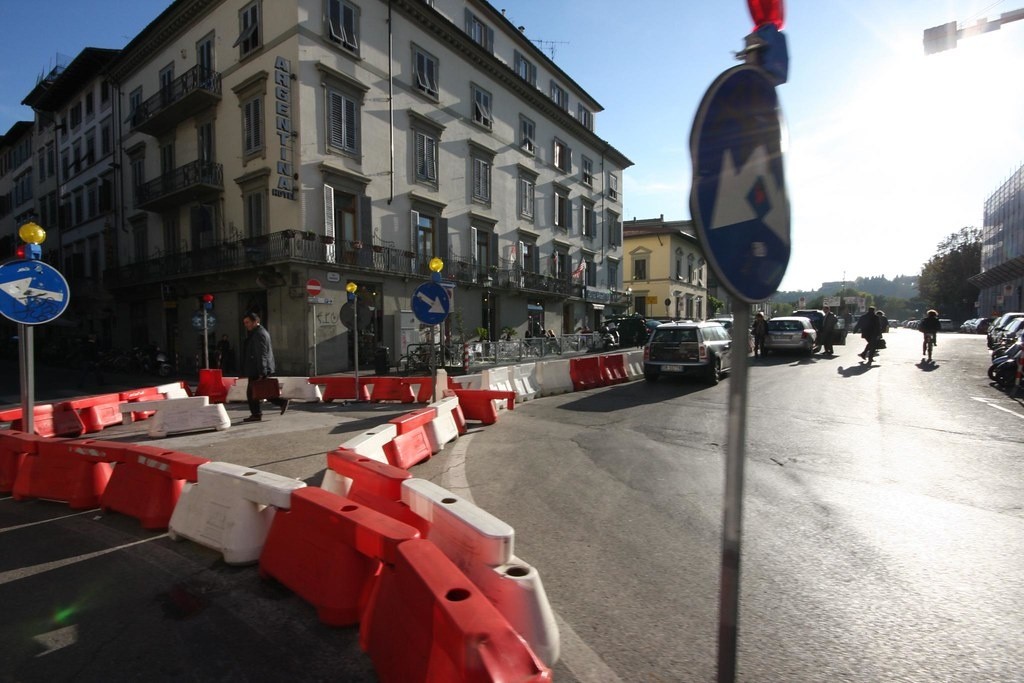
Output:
[871,339,886,349]
[252,377,281,400]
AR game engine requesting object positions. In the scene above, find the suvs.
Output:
[642,320,734,386]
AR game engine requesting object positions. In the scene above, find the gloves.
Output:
[261,369,267,377]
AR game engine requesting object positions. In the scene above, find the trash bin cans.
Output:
[374,347,391,375]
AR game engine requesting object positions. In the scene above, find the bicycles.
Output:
[533,331,563,357]
[95,345,159,379]
[396,346,463,377]
[924,331,935,361]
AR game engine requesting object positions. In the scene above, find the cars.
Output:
[764,316,849,356]
[792,309,826,328]
[887,319,953,330]
[959,313,1024,392]
[704,318,753,352]
[616,318,664,346]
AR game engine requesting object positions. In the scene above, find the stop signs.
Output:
[306,279,322,298]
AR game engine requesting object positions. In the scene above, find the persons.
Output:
[495,325,592,356]
[917,309,943,355]
[750,311,769,358]
[216,333,232,373]
[819,306,838,354]
[853,306,889,363]
[242,310,288,420]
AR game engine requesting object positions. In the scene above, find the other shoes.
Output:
[861,353,866,359]
[243,414,261,421]
[869,358,874,362]
[278,399,289,415]
